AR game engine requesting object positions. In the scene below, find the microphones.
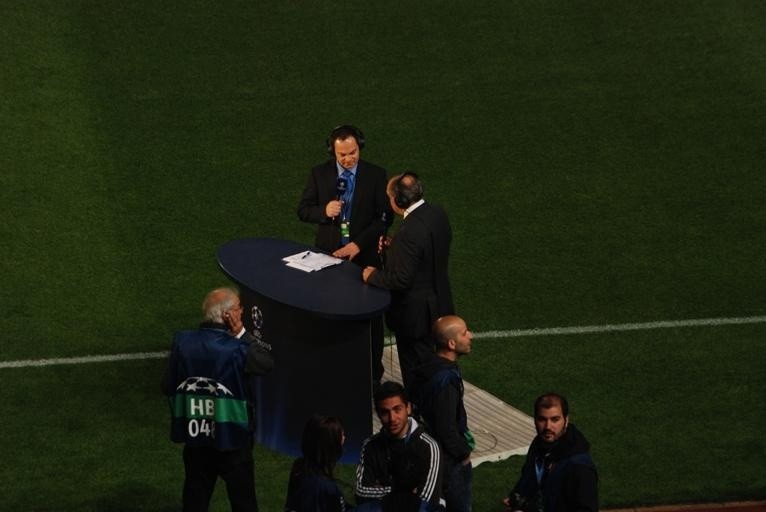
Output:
[332,178,348,220]
[381,213,388,258]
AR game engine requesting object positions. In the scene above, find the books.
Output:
[282,249,344,275]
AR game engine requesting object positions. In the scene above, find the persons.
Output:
[159,286,274,512]
[404,314,477,512]
[361,170,455,385]
[503,391,603,512]
[355,380,445,511]
[296,125,398,398]
[286,412,350,512]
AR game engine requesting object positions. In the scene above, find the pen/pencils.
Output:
[302,251,310,259]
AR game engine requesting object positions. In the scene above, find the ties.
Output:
[342,170,354,219]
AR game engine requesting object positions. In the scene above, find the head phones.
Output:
[394,171,425,209]
[325,125,365,157]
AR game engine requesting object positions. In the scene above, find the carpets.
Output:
[372,336,539,468]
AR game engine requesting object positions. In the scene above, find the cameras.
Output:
[508,493,524,512]
